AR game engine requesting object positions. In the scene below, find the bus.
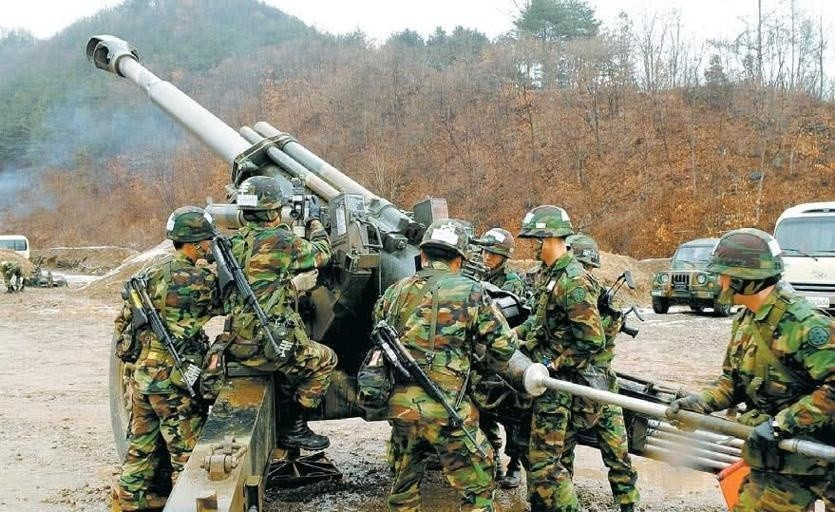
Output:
[772,202,834,317]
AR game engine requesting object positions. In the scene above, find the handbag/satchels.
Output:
[570,366,607,432]
[199,333,230,400]
[355,346,395,408]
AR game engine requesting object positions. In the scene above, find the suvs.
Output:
[650,239,745,316]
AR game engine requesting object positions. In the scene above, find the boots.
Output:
[276,417,330,451]
[500,467,522,488]
[5,285,14,293]
[491,449,504,480]
[118,489,168,512]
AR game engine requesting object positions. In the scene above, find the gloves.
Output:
[304,194,321,228]
[291,268,318,291]
[743,421,795,471]
[666,393,714,434]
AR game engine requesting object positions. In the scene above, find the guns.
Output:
[371,319,487,459]
[122,277,196,397]
[209,234,282,357]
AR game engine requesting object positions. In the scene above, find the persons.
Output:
[472,227,522,491]
[662,226,835,512]
[557,233,642,511]
[205,175,339,451]
[0,260,23,293]
[113,204,322,512]
[375,217,522,512]
[509,203,607,512]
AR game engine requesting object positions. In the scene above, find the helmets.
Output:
[419,218,470,261]
[564,234,602,269]
[704,228,784,280]
[479,228,516,259]
[517,205,575,239]
[236,175,286,211]
[165,206,221,243]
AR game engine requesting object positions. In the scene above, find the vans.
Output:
[0,234,30,260]
